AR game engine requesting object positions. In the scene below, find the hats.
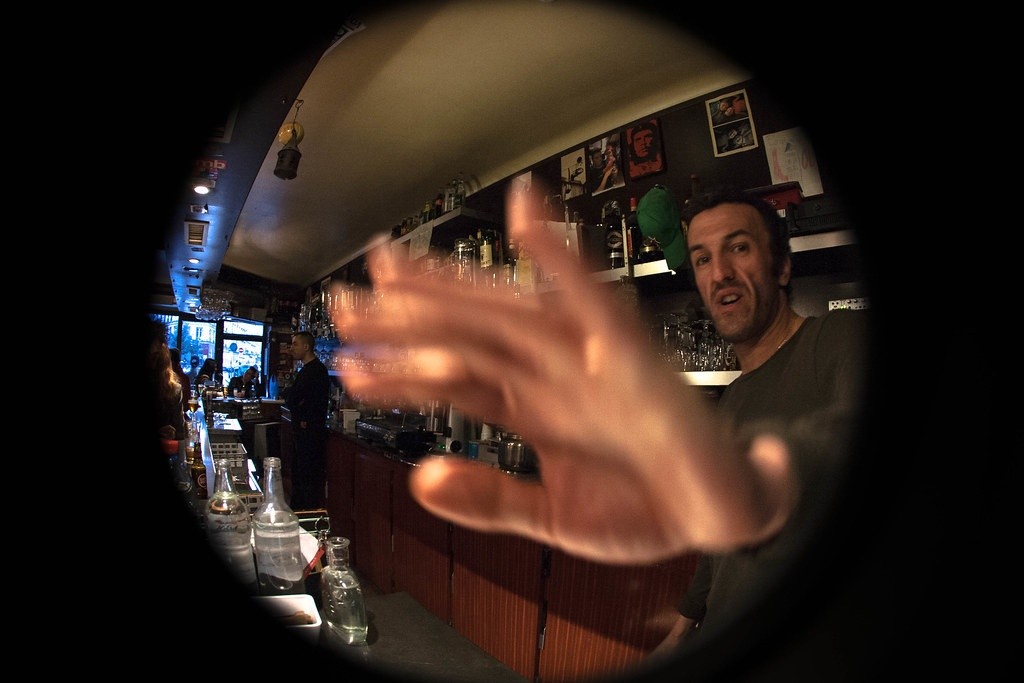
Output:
[637,184,686,269]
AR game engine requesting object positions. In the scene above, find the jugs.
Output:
[496,433,537,472]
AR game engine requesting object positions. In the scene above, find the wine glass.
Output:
[648,312,736,371]
[187,400,198,421]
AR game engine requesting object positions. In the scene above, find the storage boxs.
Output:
[209,441,249,485]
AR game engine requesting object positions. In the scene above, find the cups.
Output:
[190,392,198,401]
[198,384,204,395]
[343,412,361,429]
[186,445,194,464]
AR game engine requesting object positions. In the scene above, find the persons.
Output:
[152,319,191,440]
[280,333,330,529]
[328,181,861,658]
[625,123,665,177]
[710,95,755,153]
[587,147,613,193]
[192,359,223,395]
[186,356,199,385]
[228,367,260,398]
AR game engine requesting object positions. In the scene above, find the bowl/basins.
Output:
[252,593,322,646]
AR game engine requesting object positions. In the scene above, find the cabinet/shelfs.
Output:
[325,433,701,682]
[532,227,857,388]
[296,206,500,384]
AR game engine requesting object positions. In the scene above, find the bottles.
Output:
[241,385,245,395]
[251,385,255,399]
[253,457,307,596]
[321,537,368,646]
[191,443,208,499]
[393,172,466,234]
[204,459,252,589]
[452,228,520,302]
[295,287,384,372]
[625,197,645,263]
[606,211,624,269]
